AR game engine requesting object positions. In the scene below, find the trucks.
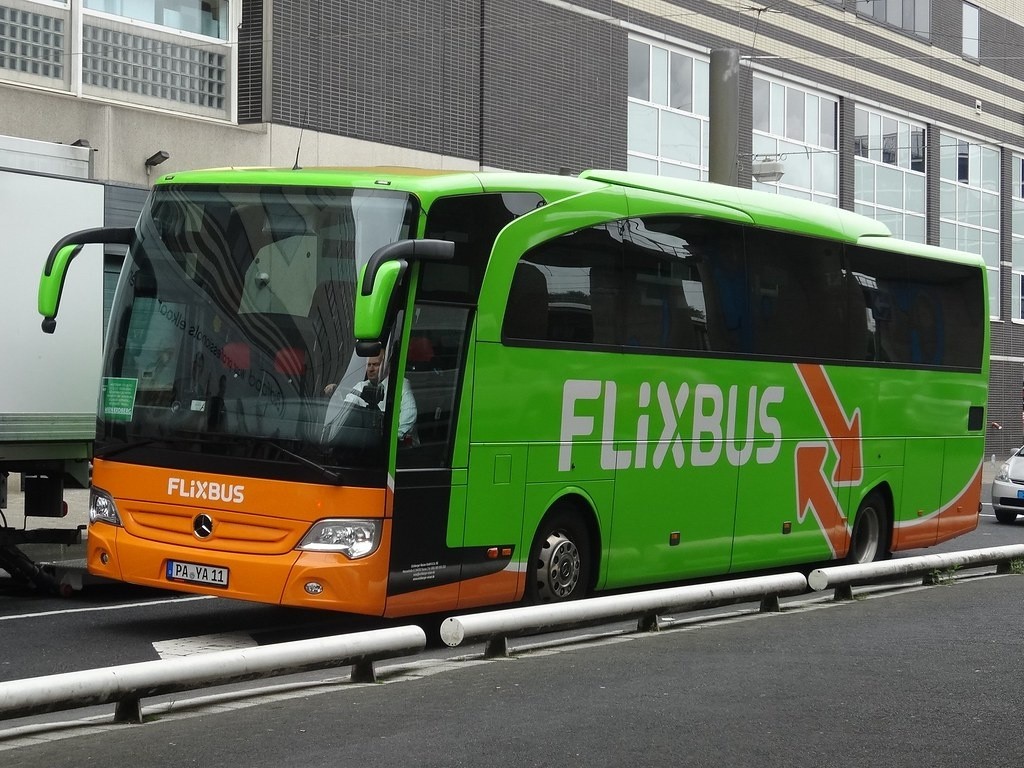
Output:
[1,133,110,517]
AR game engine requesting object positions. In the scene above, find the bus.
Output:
[35,159,992,634]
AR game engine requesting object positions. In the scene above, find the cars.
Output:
[988,444,1024,524]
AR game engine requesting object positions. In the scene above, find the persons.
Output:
[325,349,418,446]
